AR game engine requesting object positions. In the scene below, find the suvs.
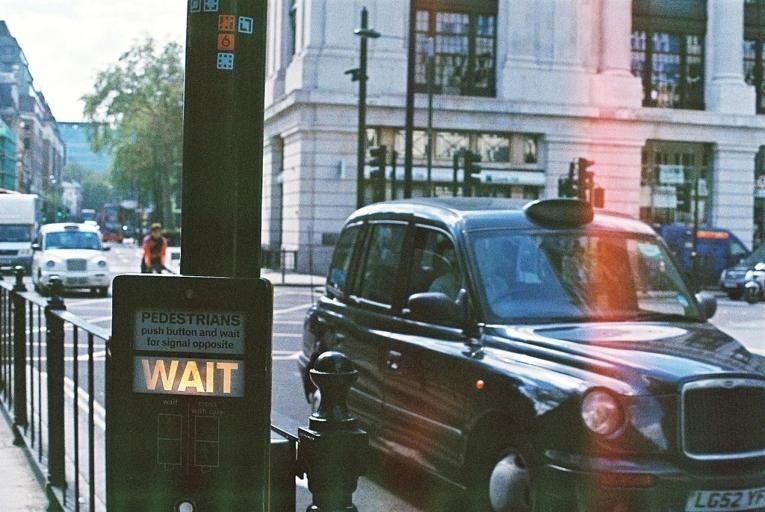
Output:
[297,192,764,512]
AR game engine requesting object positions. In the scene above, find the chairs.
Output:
[427,246,512,305]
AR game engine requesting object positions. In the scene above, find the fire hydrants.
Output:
[296,351,368,512]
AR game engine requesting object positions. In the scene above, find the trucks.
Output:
[0,192,42,272]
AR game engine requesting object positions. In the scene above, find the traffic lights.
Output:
[467,151,482,185]
[369,146,383,179]
[582,157,594,192]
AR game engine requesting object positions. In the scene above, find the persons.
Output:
[139,221,167,274]
[424,235,515,318]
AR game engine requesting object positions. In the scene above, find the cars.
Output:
[720,246,764,303]
[30,222,110,295]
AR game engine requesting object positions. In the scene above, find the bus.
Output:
[102,204,124,241]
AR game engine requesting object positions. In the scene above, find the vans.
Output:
[651,221,751,289]
